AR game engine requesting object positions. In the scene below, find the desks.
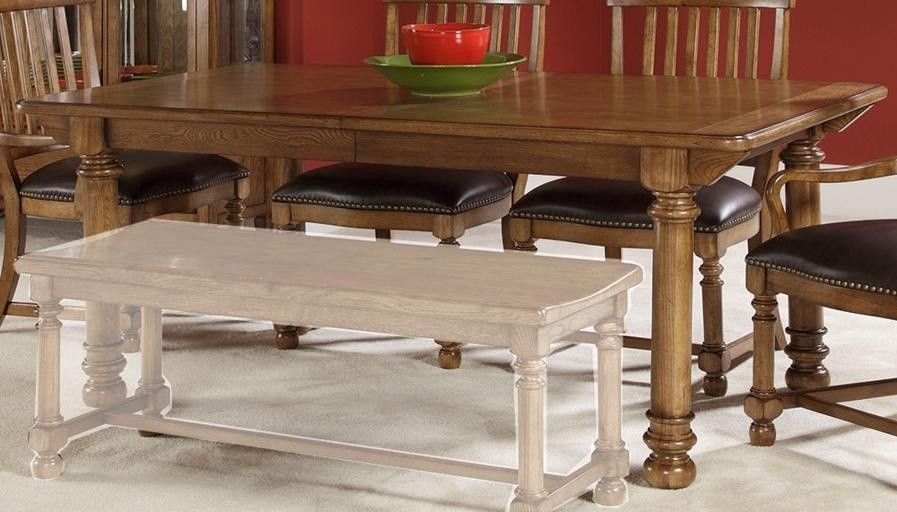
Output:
[13,57,888,493]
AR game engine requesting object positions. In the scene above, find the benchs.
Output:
[12,214,645,509]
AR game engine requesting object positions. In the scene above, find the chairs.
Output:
[270,1,551,370]
[505,0,797,400]
[743,154,897,448]
[0,1,249,328]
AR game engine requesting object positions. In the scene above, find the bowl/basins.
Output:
[402,21,491,65]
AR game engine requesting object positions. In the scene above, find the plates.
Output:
[361,51,527,98]
